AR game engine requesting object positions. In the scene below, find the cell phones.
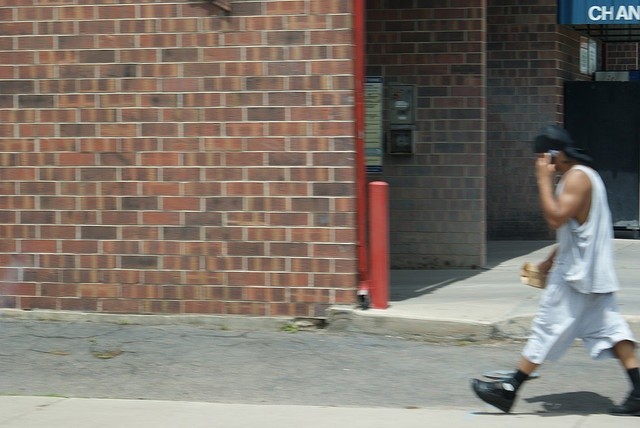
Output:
[547,146,560,168]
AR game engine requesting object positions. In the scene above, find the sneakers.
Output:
[472,377,519,413]
[609,388,639,416]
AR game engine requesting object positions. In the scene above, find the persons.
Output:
[470,123,639,418]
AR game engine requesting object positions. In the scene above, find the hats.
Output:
[534,124,593,163]
